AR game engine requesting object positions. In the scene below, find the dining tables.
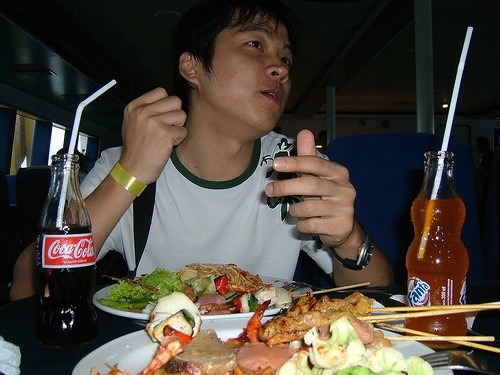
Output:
[0,282,500,375]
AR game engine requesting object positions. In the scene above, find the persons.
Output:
[10,0,392,302]
[314,129,328,152]
[472,136,494,201]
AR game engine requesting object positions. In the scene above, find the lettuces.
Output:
[96,268,216,311]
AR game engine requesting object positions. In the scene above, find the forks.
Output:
[419,349,500,375]
[281,280,392,296]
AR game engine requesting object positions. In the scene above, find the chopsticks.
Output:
[351,300,500,321]
[367,322,500,352]
[290,282,372,298]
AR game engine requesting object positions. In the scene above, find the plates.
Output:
[93,272,313,329]
[72,316,453,375]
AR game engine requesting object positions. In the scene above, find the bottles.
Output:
[36,153,95,350]
[406,151,468,350]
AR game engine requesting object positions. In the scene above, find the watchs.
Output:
[329,217,373,271]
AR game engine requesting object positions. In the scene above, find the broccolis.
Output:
[274,317,434,375]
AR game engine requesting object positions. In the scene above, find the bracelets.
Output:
[110,162,147,196]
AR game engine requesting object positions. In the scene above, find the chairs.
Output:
[0,164,53,304]
[314,131,482,285]
